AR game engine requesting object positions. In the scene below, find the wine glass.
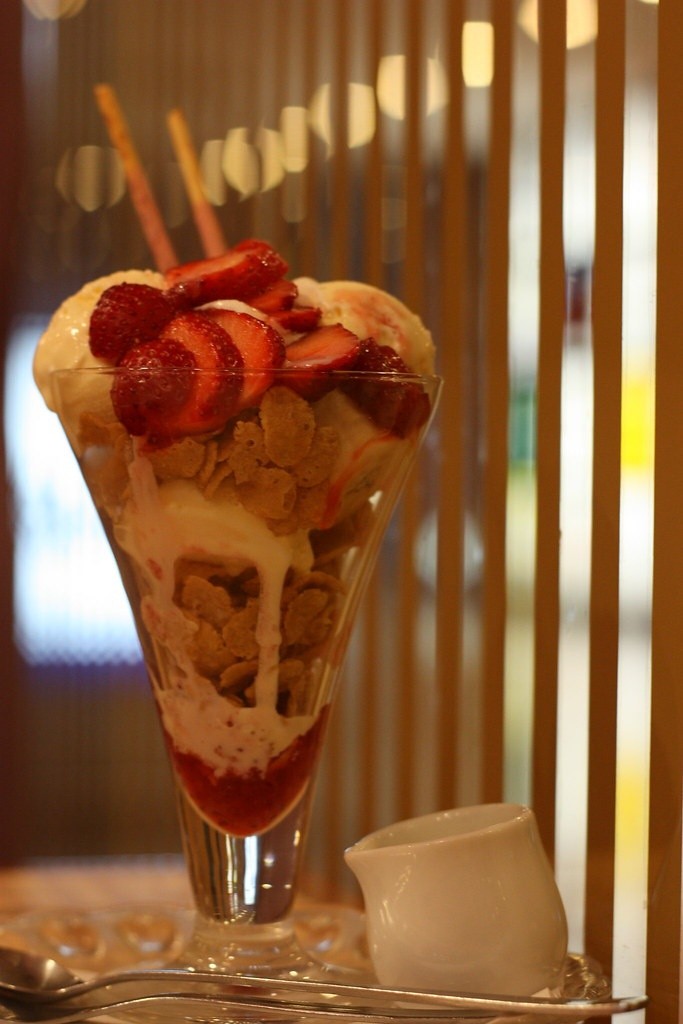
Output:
[52,367,443,1024]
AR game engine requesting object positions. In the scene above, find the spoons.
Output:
[0,946,647,1015]
[0,997,589,1024]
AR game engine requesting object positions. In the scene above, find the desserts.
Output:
[43,265,443,943]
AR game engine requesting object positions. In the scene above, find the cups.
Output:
[345,804,569,1010]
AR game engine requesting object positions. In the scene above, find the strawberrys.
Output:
[88,234,431,444]
